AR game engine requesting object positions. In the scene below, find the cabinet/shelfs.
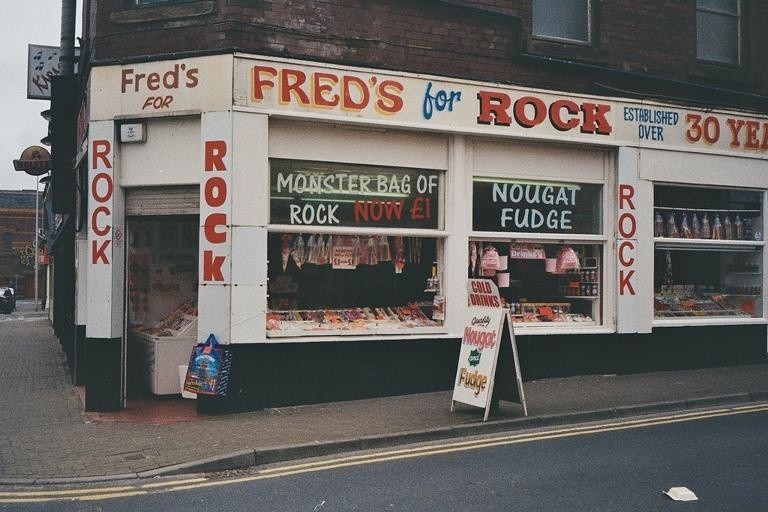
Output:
[130,293,197,397]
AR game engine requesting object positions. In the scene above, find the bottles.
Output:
[654,211,743,239]
[580,270,597,295]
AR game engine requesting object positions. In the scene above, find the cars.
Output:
[0,287,16,314]
